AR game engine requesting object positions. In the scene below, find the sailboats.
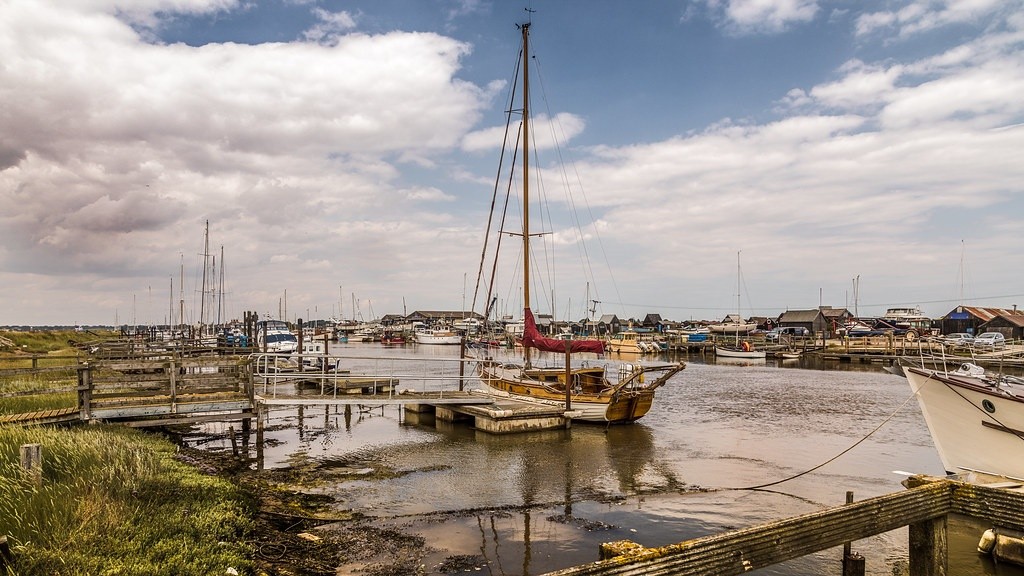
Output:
[111,219,482,373]
[464,7,687,423]
[707,251,759,334]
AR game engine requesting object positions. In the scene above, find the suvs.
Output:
[766,327,809,343]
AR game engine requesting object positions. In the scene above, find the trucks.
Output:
[412,321,426,334]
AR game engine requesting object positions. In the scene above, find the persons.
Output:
[741,340,750,352]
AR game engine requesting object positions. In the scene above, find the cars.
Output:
[945,332,976,348]
[973,331,1005,350]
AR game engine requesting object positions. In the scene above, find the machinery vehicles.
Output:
[904,319,941,342]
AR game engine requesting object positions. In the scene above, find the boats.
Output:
[605,329,659,353]
[880,336,1024,482]
